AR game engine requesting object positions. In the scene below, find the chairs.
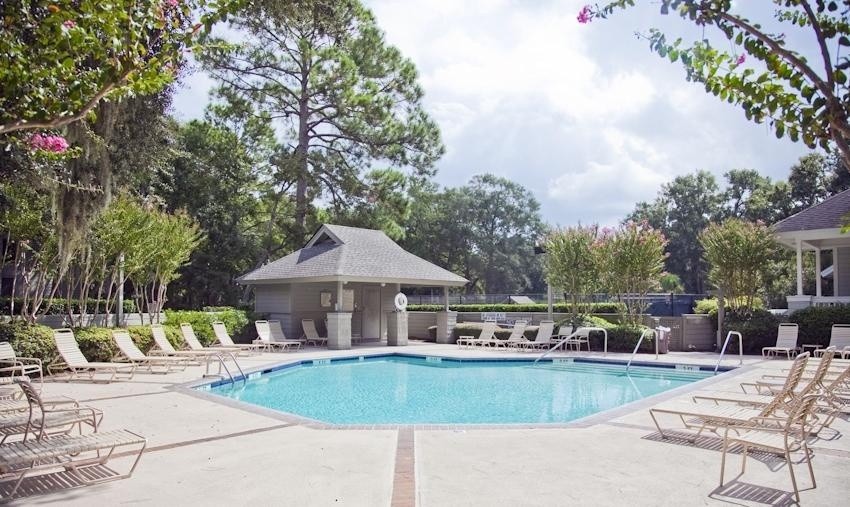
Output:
[456,319,591,352]
[0,329,147,504]
[111,318,327,374]
[648,322,849,502]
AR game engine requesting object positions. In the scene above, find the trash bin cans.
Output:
[655,326,670,354]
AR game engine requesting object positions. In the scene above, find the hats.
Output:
[394,292,408,311]
